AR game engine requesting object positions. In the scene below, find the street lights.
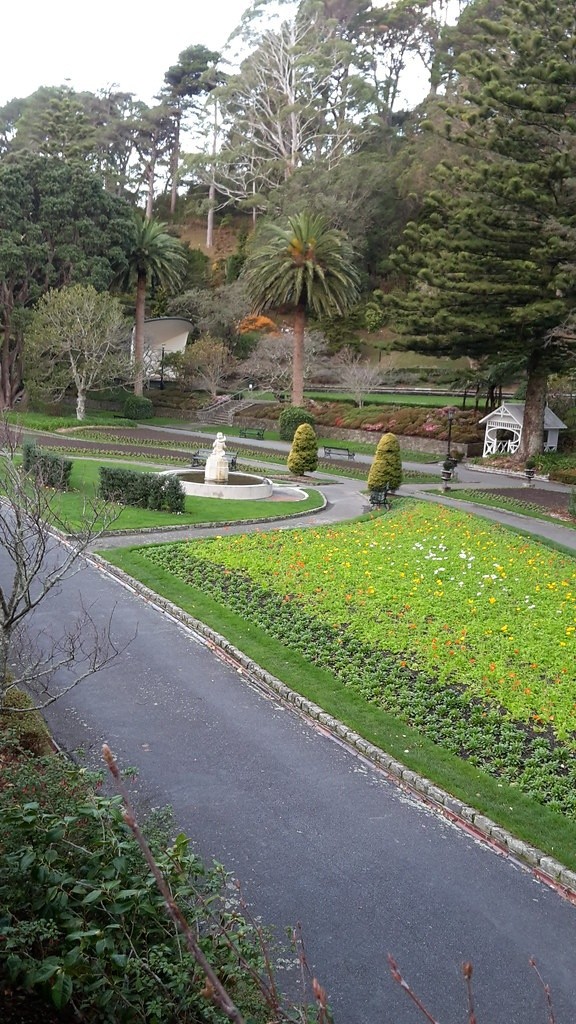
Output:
[160,343,166,390]
[446,409,454,459]
[379,330,383,363]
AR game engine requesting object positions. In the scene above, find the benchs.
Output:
[191,449,240,471]
[238,427,266,441]
[323,445,355,462]
[369,481,390,510]
